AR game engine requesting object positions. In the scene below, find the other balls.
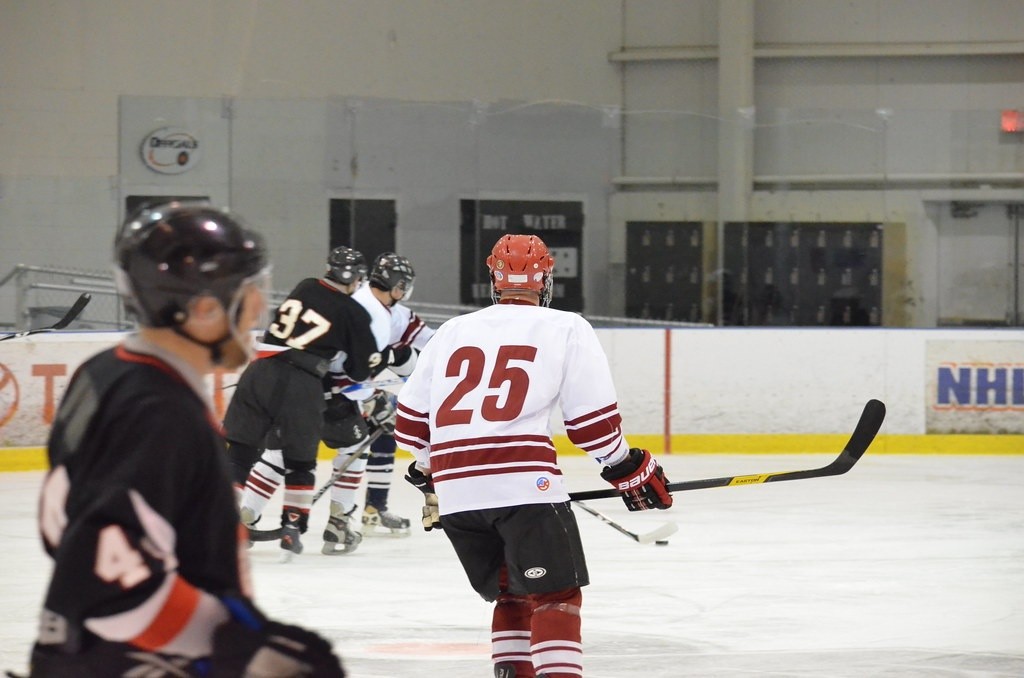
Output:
[655,540,669,546]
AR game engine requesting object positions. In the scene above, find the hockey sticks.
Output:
[0,291,92,342]
[339,375,409,394]
[247,426,386,542]
[572,499,680,546]
[567,397,886,502]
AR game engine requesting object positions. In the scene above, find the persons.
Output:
[216,243,382,554]
[392,233,673,678]
[244,253,438,553]
[28,195,346,678]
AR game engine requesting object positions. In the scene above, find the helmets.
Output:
[487,234,553,289]
[370,253,415,291]
[327,246,367,283]
[112,196,269,328]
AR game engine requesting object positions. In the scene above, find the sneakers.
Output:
[321,499,363,556]
[360,507,409,538]
[278,505,303,562]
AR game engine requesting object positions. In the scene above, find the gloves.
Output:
[404,461,443,531]
[364,390,397,435]
[600,447,673,513]
[194,611,345,677]
